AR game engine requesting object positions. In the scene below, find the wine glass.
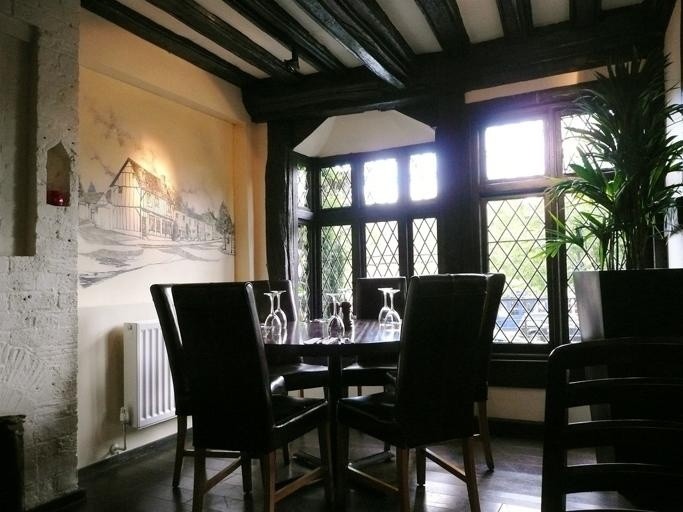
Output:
[264,290,288,337]
[326,293,345,337]
[377,287,401,332]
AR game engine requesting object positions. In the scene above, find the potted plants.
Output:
[536,37,683,512]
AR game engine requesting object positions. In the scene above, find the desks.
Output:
[260,319,403,510]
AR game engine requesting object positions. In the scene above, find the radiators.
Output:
[119,319,177,430]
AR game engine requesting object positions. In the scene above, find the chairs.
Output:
[149,282,289,488]
[340,277,408,453]
[385,274,504,471]
[244,280,330,461]
[172,283,334,510]
[336,274,489,510]
[542,338,683,512]
